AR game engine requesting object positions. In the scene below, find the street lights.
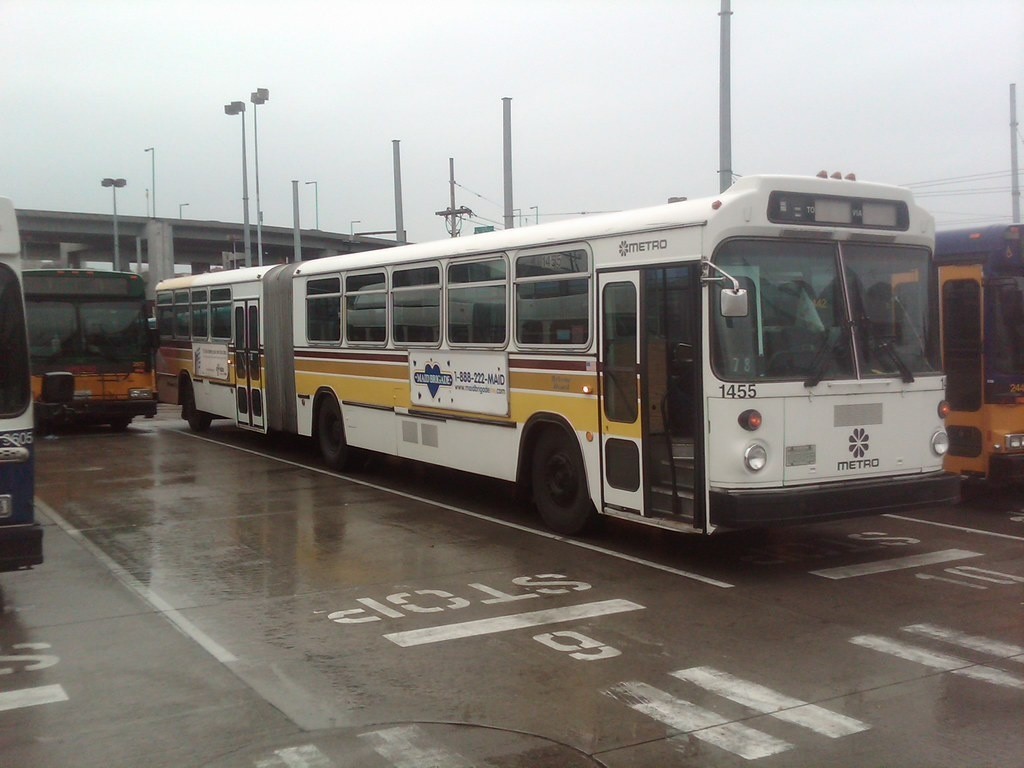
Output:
[350,221,361,235]
[101,178,127,272]
[224,102,252,269]
[144,148,156,217]
[179,203,189,220]
[250,87,269,266]
[512,209,521,227]
[530,206,538,225]
[305,181,318,230]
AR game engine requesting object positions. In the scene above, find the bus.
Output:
[152,172,961,543]
[0,198,44,577]
[340,269,608,347]
[605,224,1024,478]
[23,266,158,430]
